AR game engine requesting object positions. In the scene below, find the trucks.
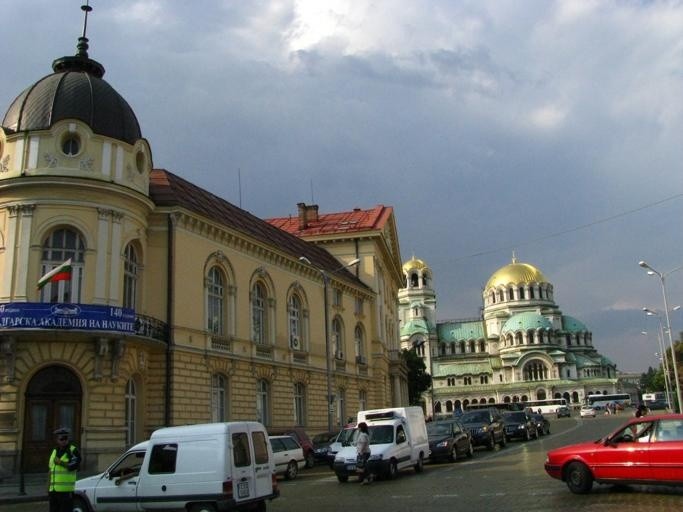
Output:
[334,406,431,482]
[72,420,281,512]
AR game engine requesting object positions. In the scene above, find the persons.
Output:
[356,422,375,485]
[606,402,623,415]
[636,405,647,417]
[631,424,656,443]
[397,430,406,442]
[114,472,138,481]
[47,427,81,511]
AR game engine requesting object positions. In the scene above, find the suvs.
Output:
[270,429,315,469]
[459,406,508,450]
[266,435,308,480]
[327,422,360,470]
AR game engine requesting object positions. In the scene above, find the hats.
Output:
[54,427,71,436]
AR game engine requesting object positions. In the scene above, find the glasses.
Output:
[60,437,68,441]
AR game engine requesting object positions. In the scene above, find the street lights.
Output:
[640,303,681,412]
[638,260,683,414]
[300,256,362,432]
[654,350,667,361]
[641,331,672,410]
[414,316,442,420]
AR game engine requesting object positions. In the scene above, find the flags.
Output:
[36,258,72,291]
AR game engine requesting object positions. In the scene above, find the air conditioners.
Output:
[292,335,300,351]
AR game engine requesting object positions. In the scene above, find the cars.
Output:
[544,414,683,496]
[312,431,335,463]
[424,417,475,463]
[555,407,571,418]
[580,405,597,418]
[529,413,550,436]
[501,411,539,441]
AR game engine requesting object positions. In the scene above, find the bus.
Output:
[583,393,632,410]
[641,391,676,410]
[524,398,569,414]
